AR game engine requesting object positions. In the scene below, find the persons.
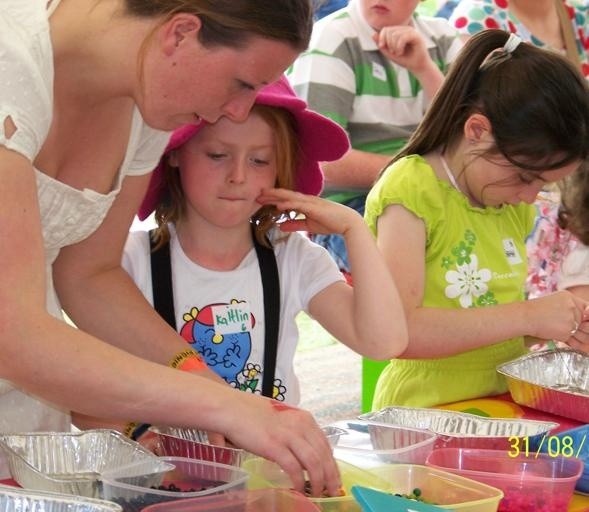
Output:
[0,0,343,501]
[447,0,589,353]
[70,69,408,457]
[363,28,589,415]
[554,155,589,246]
[281,0,466,278]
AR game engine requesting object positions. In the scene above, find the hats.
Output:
[137,72,349,220]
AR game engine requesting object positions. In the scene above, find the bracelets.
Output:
[169,348,207,372]
[123,421,153,439]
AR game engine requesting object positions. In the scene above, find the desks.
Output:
[0,390,589,512]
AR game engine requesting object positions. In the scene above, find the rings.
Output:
[571,320,579,334]
